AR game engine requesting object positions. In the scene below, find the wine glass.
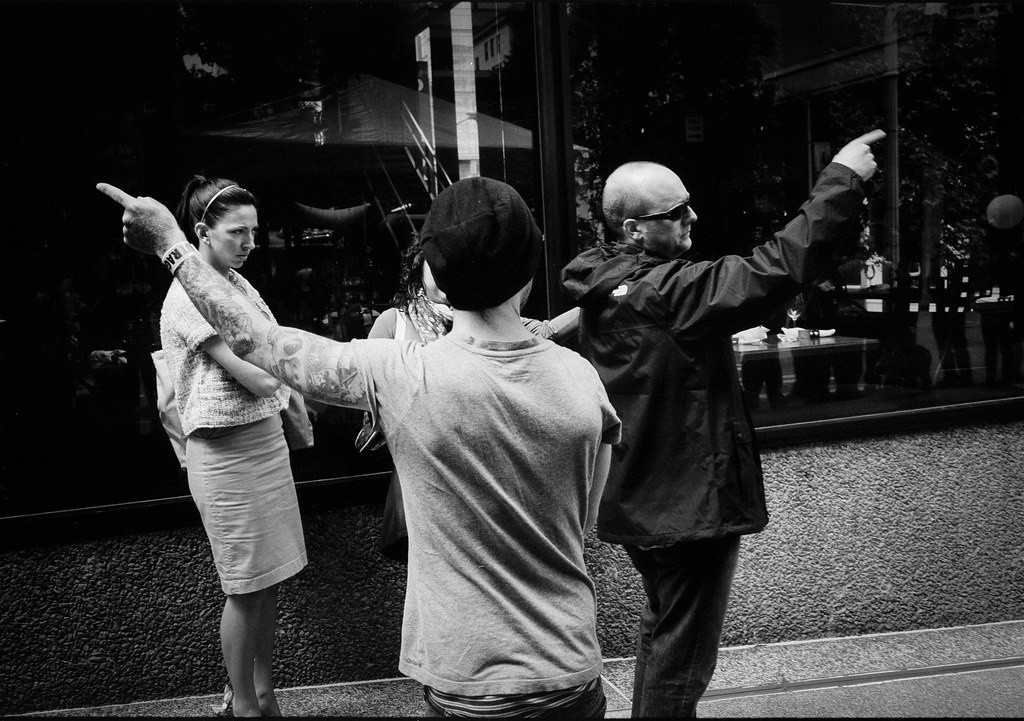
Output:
[908,261,921,288]
[787,291,805,328]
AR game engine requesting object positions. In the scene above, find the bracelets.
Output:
[162,241,201,276]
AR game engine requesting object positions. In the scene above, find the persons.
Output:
[94,176,622,720]
[157,176,317,720]
[368,235,579,560]
[559,129,888,721]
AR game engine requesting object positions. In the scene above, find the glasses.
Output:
[632,200,692,222]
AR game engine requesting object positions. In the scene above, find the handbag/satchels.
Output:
[151,348,313,472]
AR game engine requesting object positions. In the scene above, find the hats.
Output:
[419,176,543,312]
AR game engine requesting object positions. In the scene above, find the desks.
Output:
[733,335,881,406]
